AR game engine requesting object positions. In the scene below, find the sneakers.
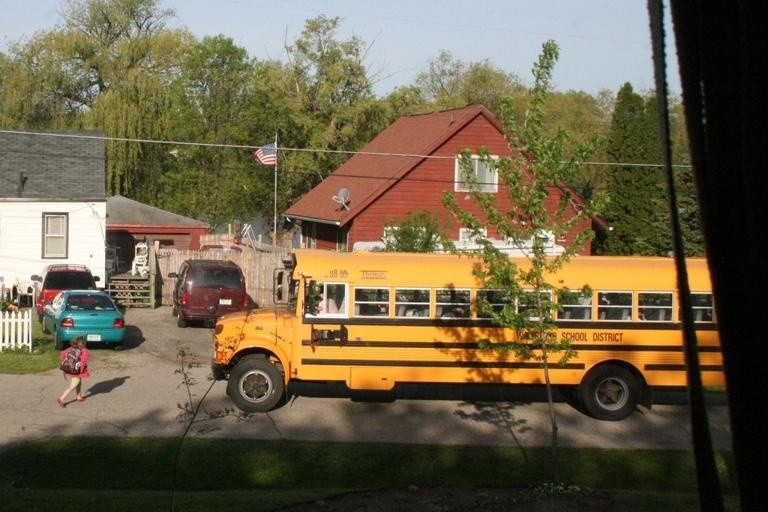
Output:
[57,396,85,408]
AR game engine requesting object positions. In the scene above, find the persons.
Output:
[310,285,340,314]
[55,335,91,407]
[627,307,647,322]
[700,294,713,320]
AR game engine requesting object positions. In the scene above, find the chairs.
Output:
[131,241,149,280]
[338,294,704,322]
[17,281,35,308]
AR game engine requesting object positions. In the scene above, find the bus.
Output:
[212,250,727,413]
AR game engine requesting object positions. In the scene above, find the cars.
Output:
[31,264,126,350]
[168,259,248,328]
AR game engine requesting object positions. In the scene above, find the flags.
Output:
[254,142,277,165]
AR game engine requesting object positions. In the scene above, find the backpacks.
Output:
[60,347,82,375]
